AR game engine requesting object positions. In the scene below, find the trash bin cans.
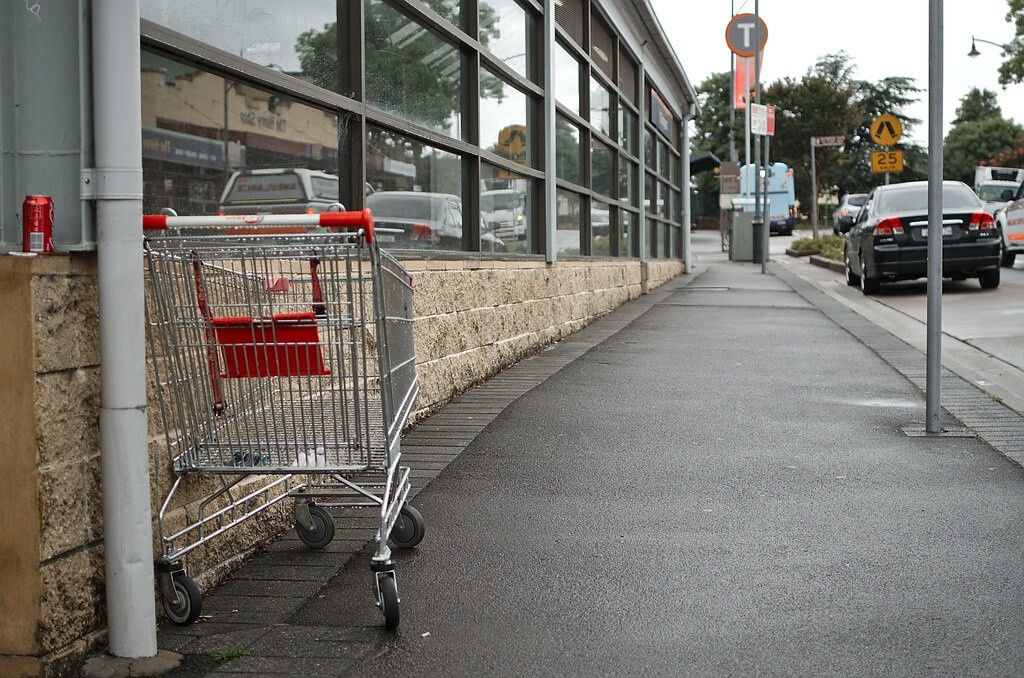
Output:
[729,197,771,263]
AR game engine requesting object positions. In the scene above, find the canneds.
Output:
[20,193,56,256]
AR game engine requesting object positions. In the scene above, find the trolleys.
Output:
[139,205,428,631]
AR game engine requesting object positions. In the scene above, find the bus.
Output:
[973,166,1024,222]
[738,162,799,236]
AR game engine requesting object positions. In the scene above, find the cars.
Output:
[844,178,1005,291]
[994,173,1024,268]
[829,192,869,232]
[214,166,629,255]
